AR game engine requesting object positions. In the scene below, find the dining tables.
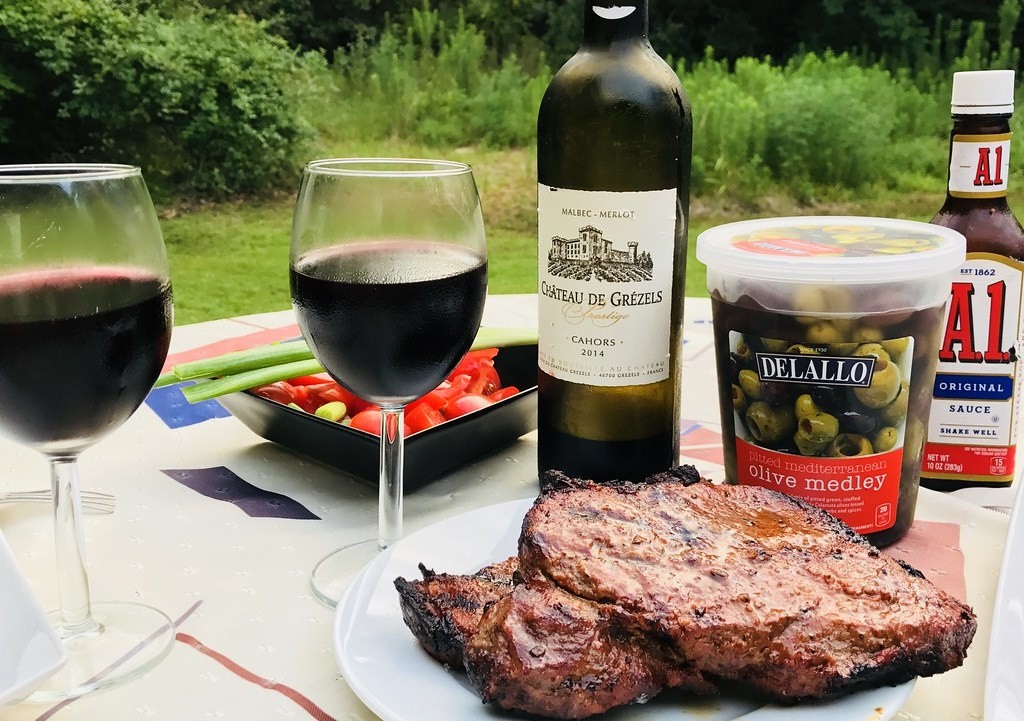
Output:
[1,294,1022,720]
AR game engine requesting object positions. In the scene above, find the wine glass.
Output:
[288,160,489,607]
[1,164,174,691]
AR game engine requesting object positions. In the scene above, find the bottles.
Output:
[918,70,1023,493]
[535,1,693,487]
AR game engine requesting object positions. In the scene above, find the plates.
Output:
[334,498,918,721]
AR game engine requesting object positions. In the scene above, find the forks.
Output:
[1,486,116,514]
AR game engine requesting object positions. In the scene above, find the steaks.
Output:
[394,466,978,721]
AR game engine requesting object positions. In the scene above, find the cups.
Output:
[696,217,966,550]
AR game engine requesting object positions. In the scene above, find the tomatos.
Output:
[253,348,519,439]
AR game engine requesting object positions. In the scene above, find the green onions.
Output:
[155,328,539,403]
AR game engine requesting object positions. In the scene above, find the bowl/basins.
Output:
[195,335,538,497]
